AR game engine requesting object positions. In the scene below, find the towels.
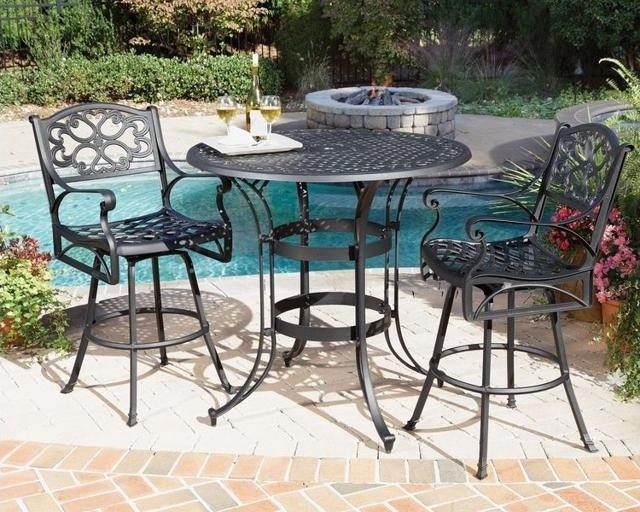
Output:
[219,126,257,146]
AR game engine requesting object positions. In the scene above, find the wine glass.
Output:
[259,95,282,146]
[216,95,240,146]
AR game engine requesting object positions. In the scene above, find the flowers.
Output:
[546,206,636,304]
[0,203,83,366]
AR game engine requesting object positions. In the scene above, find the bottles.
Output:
[245,64,265,144]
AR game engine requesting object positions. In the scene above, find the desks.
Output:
[184,130,471,454]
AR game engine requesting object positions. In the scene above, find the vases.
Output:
[0,318,29,347]
[601,297,626,329]
[563,279,600,325]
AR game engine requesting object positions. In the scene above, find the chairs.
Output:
[401,121,633,479]
[29,104,237,427]
